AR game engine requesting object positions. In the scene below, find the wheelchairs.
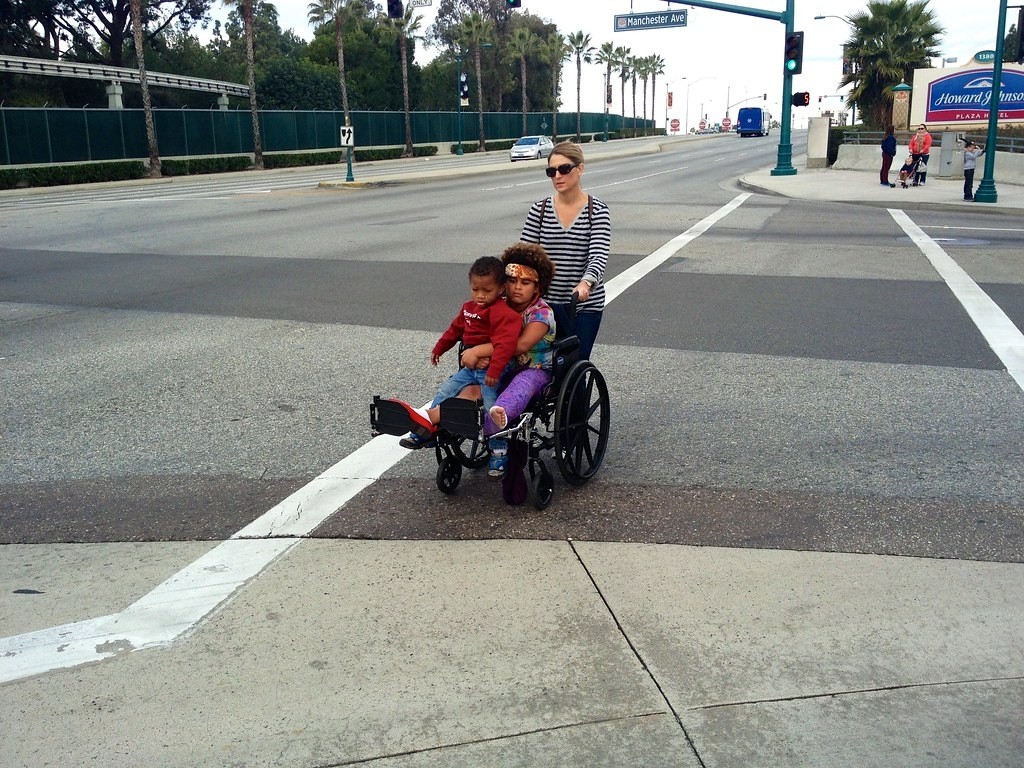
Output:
[372,294,610,513]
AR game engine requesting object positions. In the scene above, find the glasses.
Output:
[918,128,924,130]
[546,164,579,177]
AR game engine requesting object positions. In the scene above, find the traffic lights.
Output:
[840,96,843,102]
[819,96,822,102]
[794,92,809,106]
[387,0,402,18]
[784,31,804,75]
[506,0,521,8]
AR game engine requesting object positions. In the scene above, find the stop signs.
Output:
[699,122,705,129]
[671,119,680,128]
[722,118,731,126]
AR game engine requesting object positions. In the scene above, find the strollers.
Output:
[889,151,929,189]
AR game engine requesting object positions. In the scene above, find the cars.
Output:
[695,129,717,135]
[509,135,554,162]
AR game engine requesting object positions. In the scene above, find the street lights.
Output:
[665,77,687,136]
[700,99,713,120]
[454,42,492,155]
[602,73,608,142]
[816,14,858,127]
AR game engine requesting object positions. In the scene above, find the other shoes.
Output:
[965,197,975,201]
[881,182,891,186]
[919,181,925,186]
[913,181,918,186]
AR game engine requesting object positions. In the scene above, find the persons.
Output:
[389,242,557,437]
[519,141,610,460]
[900,157,914,181]
[880,126,897,186]
[399,257,523,483]
[963,142,986,202]
[909,124,932,186]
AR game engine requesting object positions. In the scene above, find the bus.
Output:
[736,106,772,137]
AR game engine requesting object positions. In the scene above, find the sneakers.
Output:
[487,452,509,481]
[400,437,436,449]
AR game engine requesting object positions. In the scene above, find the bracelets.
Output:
[584,280,591,287]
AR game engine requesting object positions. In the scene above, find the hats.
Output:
[966,142,975,147]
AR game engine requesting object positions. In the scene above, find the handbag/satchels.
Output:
[917,162,927,172]
